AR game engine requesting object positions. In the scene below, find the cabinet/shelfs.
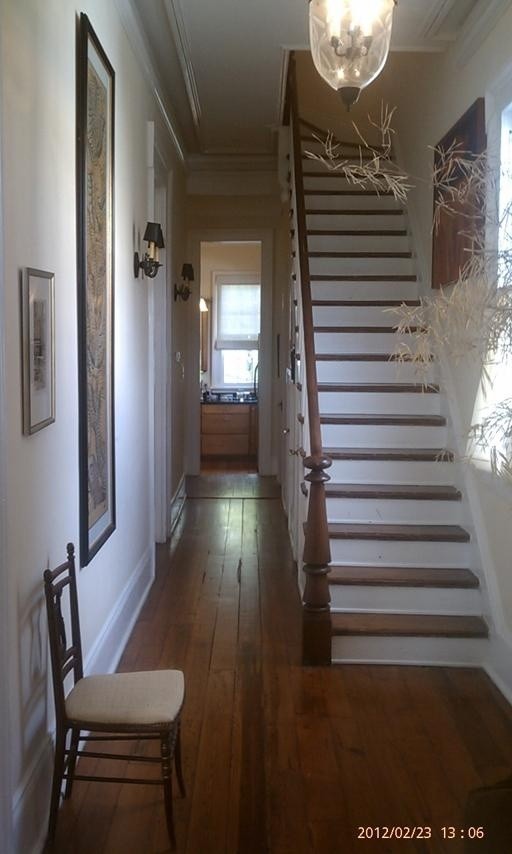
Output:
[201,403,253,457]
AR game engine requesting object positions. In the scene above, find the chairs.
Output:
[41,542,190,850]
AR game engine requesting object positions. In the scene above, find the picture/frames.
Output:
[21,266,57,438]
[72,9,120,569]
[430,93,487,292]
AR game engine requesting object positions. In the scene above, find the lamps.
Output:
[134,221,165,280]
[174,263,197,304]
[306,0,402,116]
